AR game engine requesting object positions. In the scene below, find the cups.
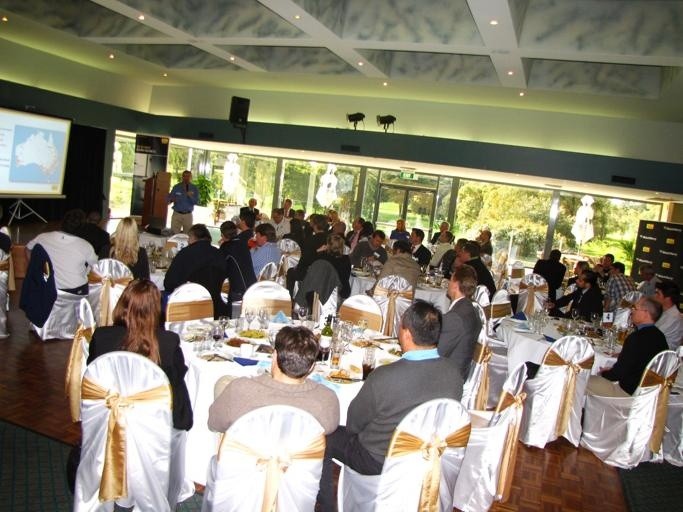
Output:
[258,306,270,329]
[435,274,444,288]
[528,309,550,335]
[194,332,203,351]
[605,327,617,353]
[364,348,375,360]
[211,327,223,347]
[245,307,256,332]
[306,315,315,331]
[318,338,331,366]
[149,240,154,248]
[240,343,253,357]
[218,316,230,337]
[357,319,368,339]
[362,357,376,381]
[298,307,308,326]
[572,309,579,323]
[234,316,244,334]
[150,261,157,273]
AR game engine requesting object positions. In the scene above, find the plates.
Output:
[232,352,260,361]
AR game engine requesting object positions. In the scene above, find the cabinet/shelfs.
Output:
[143,171,172,234]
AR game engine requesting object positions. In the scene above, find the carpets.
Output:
[618,461,683,511]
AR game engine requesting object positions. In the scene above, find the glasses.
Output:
[632,307,648,313]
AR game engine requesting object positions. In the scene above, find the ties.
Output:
[285,210,287,218]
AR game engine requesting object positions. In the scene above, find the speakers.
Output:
[229,95,251,129]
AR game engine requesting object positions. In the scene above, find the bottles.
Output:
[436,263,444,275]
[318,314,332,361]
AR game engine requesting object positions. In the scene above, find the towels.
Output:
[511,311,526,320]
[516,323,529,329]
[224,333,228,338]
[543,334,556,342]
[259,361,272,366]
[234,357,259,366]
[272,311,288,323]
[312,374,321,382]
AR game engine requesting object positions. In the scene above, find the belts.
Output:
[176,211,192,214]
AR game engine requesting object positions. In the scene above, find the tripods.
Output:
[6,198,49,246]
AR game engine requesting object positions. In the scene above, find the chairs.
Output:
[73,351,187,512]
[662,393,683,464]
[580,351,682,468]
[202,405,326,512]
[165,283,213,336]
[461,301,508,410]
[511,261,526,287]
[372,274,413,338]
[241,281,291,320]
[517,336,595,448]
[490,290,512,319]
[159,242,177,267]
[453,363,528,512]
[472,285,490,321]
[259,238,301,287]
[612,291,645,329]
[517,273,548,315]
[384,239,398,259]
[295,261,338,320]
[488,249,508,290]
[332,398,471,511]
[166,234,190,252]
[65,298,96,422]
[0,227,15,339]
[87,259,135,326]
[338,295,382,335]
[27,245,102,342]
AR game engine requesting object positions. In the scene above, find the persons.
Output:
[367,239,420,297]
[604,262,638,311]
[438,263,482,386]
[476,230,494,257]
[0,204,14,261]
[296,209,313,237]
[26,212,100,294]
[213,220,257,302]
[298,234,351,298]
[637,263,662,295]
[310,215,330,251]
[568,261,589,285]
[324,298,464,508]
[76,205,109,254]
[164,223,219,292]
[109,216,150,280]
[237,212,256,240]
[209,322,340,435]
[350,230,388,273]
[455,242,497,301]
[280,199,296,219]
[410,228,433,266]
[534,250,566,309]
[284,218,310,250]
[345,216,365,244]
[584,295,669,397]
[352,222,373,250]
[168,170,200,235]
[429,229,454,267]
[240,199,260,229]
[268,209,291,240]
[431,221,455,246]
[84,279,192,430]
[391,220,410,240]
[652,281,682,351]
[595,254,614,281]
[547,270,604,323]
[250,223,283,277]
[439,238,468,279]
